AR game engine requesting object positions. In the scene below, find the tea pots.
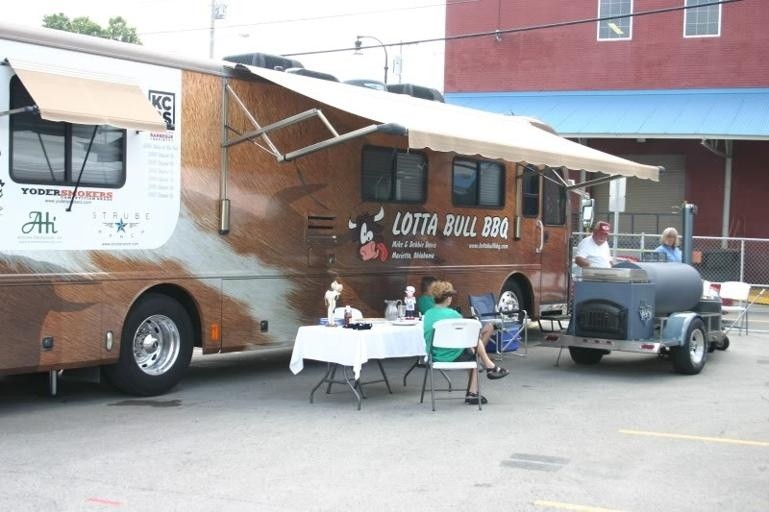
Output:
[383,299,403,322]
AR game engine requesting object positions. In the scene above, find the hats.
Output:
[592,221,612,235]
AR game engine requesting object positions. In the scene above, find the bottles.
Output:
[343,304,352,327]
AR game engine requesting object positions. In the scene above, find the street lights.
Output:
[354,33,390,84]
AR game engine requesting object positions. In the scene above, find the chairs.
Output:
[421,319,482,411]
[719,281,750,336]
[468,293,528,360]
[326,307,392,399]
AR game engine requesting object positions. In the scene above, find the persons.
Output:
[576,221,616,268]
[424,281,510,404]
[405,284,416,319]
[658,227,682,262]
[418,276,494,354]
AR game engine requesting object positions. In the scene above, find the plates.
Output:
[391,320,418,326]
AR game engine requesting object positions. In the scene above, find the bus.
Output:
[0,17,597,397]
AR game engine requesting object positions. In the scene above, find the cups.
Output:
[398,305,405,321]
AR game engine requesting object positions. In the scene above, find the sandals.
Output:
[486,367,510,380]
[465,393,487,404]
